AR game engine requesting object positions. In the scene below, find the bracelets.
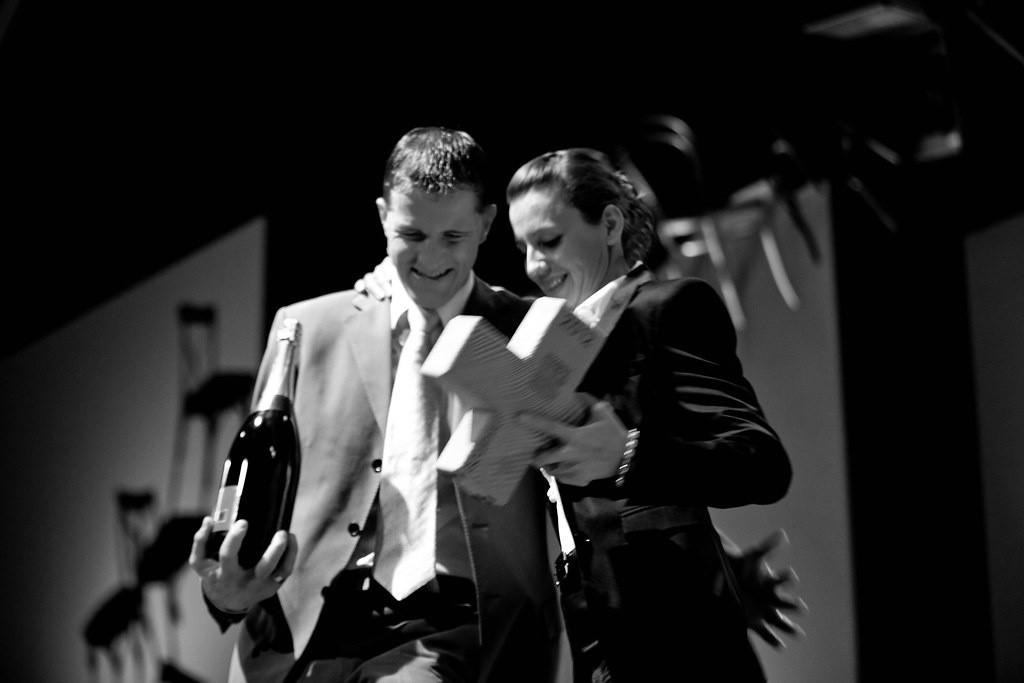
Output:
[616,427,641,486]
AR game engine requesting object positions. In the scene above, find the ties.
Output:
[370,308,441,605]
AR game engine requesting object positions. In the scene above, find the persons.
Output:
[357,147,794,682]
[190,130,806,683]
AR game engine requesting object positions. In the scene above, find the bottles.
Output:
[204,316,302,574]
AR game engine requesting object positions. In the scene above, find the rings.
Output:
[272,573,285,586]
